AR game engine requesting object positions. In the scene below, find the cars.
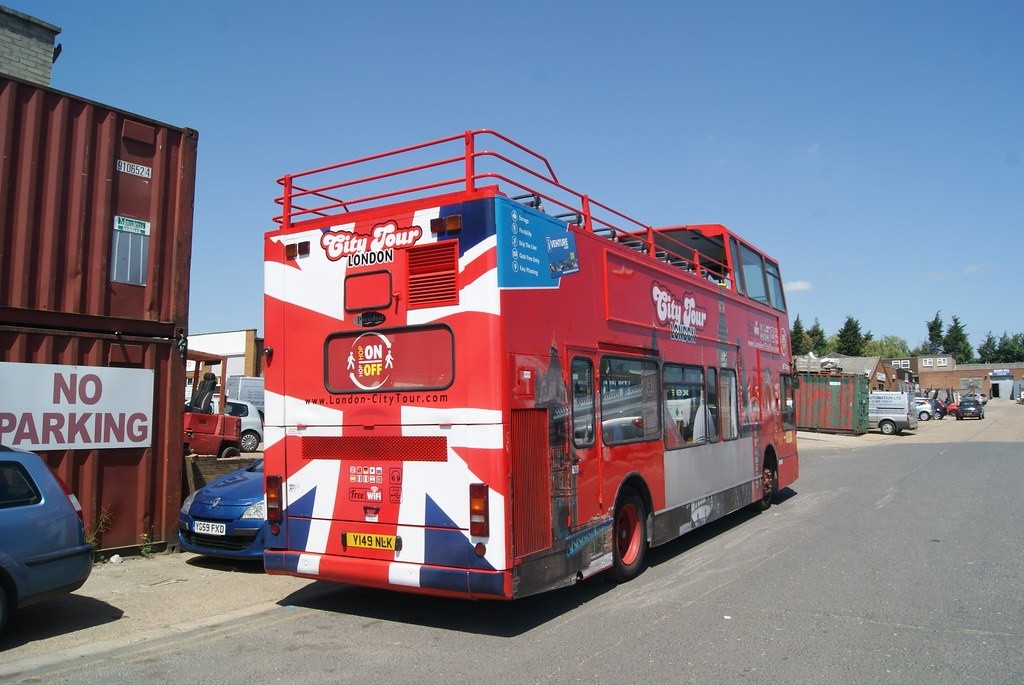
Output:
[179,459,263,561]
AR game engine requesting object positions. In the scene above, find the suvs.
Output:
[0,446,96,637]
[963,394,987,404]
[213,397,263,452]
[914,396,959,421]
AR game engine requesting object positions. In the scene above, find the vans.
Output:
[869,390,918,435]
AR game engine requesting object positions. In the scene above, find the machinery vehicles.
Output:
[183,348,241,458]
[923,388,959,416]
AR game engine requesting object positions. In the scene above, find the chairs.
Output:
[511,193,708,279]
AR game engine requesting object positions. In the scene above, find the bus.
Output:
[264,128,799,602]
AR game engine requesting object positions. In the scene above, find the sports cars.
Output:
[956,400,985,420]
